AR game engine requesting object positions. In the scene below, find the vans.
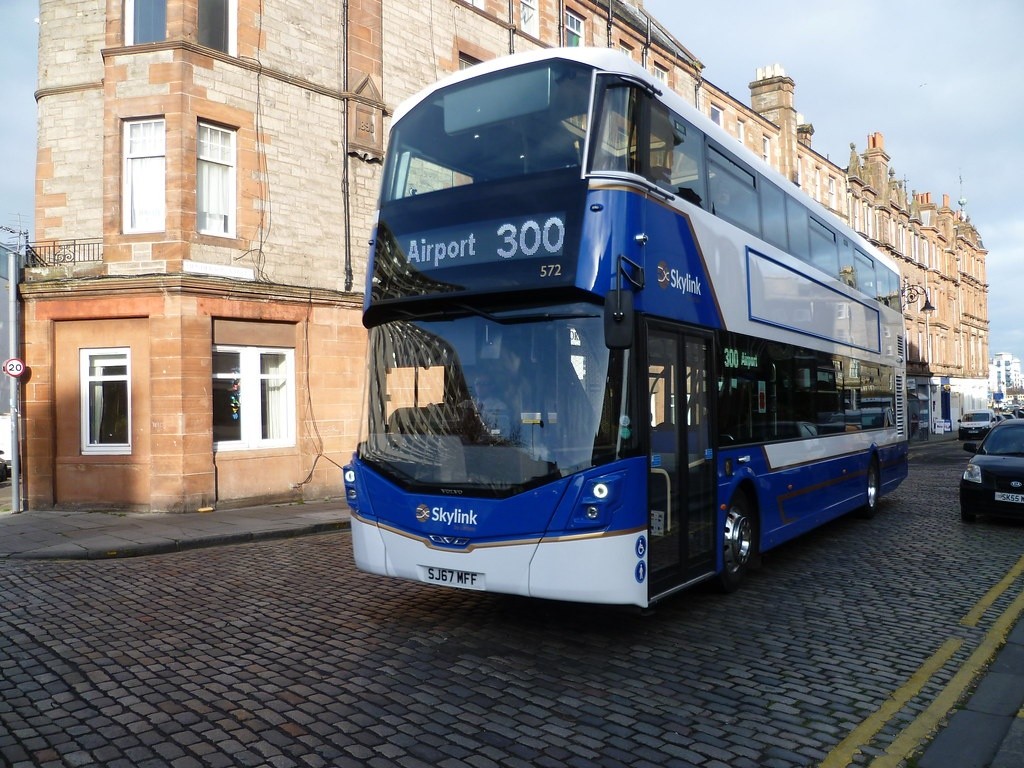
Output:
[958,410,997,440]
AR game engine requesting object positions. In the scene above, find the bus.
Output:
[338,47,912,611]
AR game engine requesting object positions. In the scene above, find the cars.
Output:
[959,418,1024,522]
[995,406,1024,422]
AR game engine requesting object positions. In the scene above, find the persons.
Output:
[426,372,511,446]
[910,412,922,439]
